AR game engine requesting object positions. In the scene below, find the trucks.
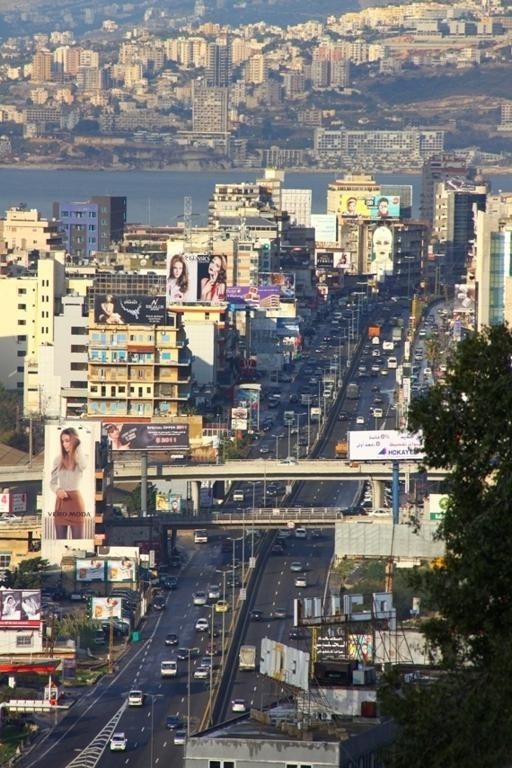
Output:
[346,380,360,400]
[334,439,348,458]
[239,645,256,672]
[233,490,244,501]
[160,660,178,679]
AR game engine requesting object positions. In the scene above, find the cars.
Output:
[369,385,383,419]
[251,608,287,621]
[104,587,140,639]
[206,642,218,656]
[177,649,188,661]
[164,575,178,590]
[165,633,178,646]
[194,657,214,680]
[266,485,277,496]
[291,561,307,588]
[283,358,325,448]
[357,317,404,377]
[166,715,187,746]
[315,312,344,354]
[313,531,322,538]
[260,445,270,454]
[231,699,246,712]
[152,597,166,612]
[110,732,128,751]
[272,528,291,555]
[196,618,219,638]
[339,412,364,425]
[194,585,229,613]
[414,314,438,369]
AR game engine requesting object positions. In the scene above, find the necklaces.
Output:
[119,297,140,320]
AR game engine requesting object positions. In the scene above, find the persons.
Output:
[105,598,114,609]
[119,557,131,578]
[22,595,40,620]
[344,196,362,219]
[374,197,391,218]
[98,295,124,324]
[103,422,131,449]
[49,426,84,538]
[166,254,189,306]
[0,595,20,620]
[200,255,227,302]
[89,560,98,573]
[369,226,393,272]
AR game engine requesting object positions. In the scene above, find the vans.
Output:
[128,690,143,708]
[295,528,307,539]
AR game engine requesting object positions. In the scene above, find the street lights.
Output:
[272,434,285,460]
[215,537,243,631]
[331,308,360,379]
[177,647,200,738]
[201,604,218,689]
[237,481,261,588]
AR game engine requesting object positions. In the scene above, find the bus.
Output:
[193,529,208,544]
